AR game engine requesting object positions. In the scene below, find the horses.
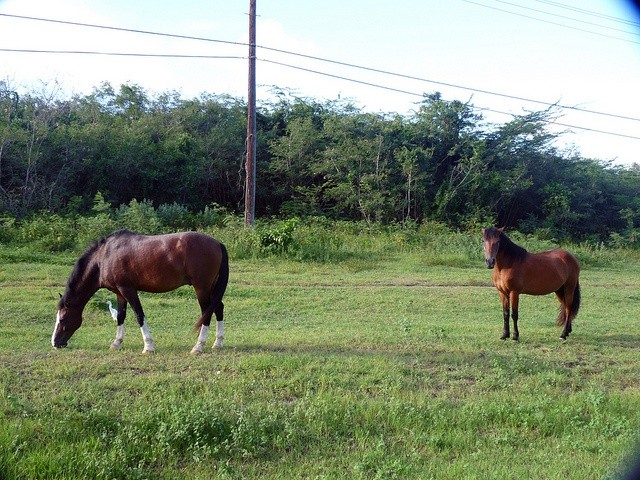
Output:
[51,229,228,354]
[480,226,580,343]
